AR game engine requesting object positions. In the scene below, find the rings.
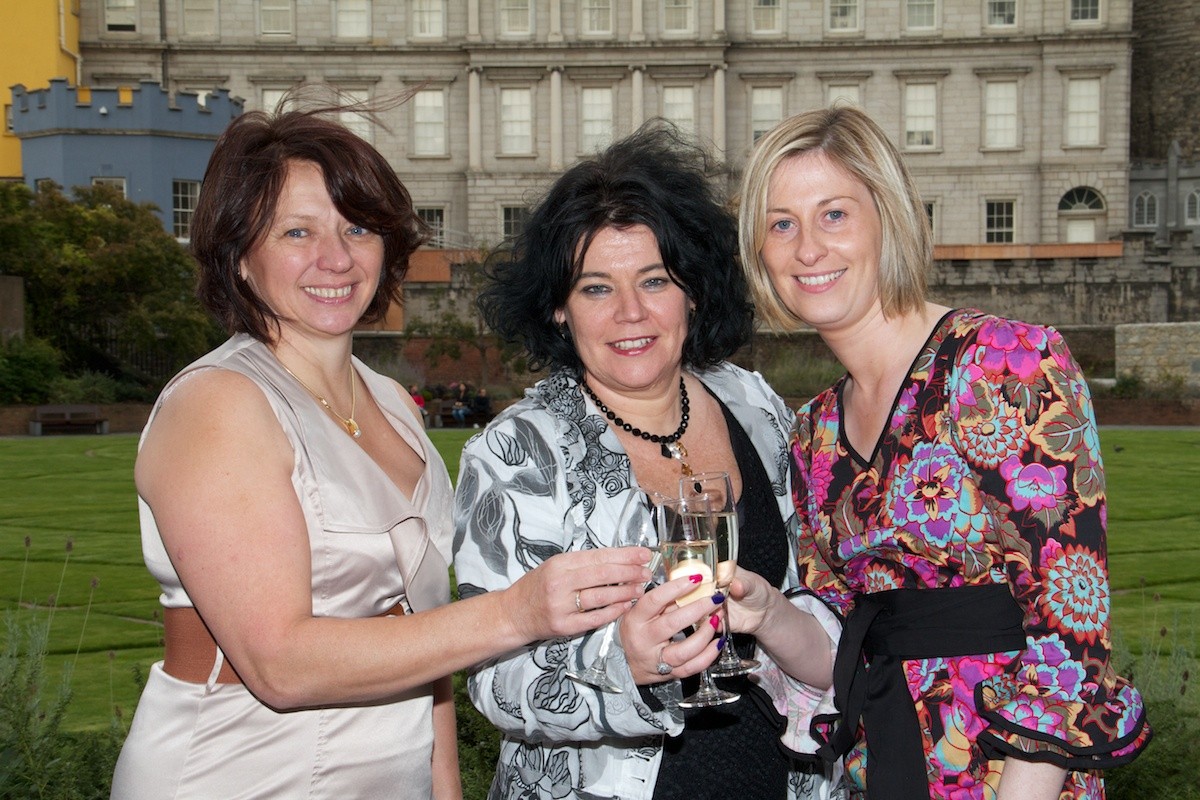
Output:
[576,591,584,611]
[656,649,672,674]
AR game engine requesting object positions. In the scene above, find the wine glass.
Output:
[563,486,681,694]
[679,472,762,678]
[657,495,742,707]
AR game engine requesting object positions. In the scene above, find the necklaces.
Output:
[581,375,702,495]
[282,361,361,438]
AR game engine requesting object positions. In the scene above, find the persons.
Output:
[738,107,1155,800]
[110,117,653,800]
[451,383,472,427]
[407,383,429,416]
[471,387,496,427]
[449,116,832,800]
